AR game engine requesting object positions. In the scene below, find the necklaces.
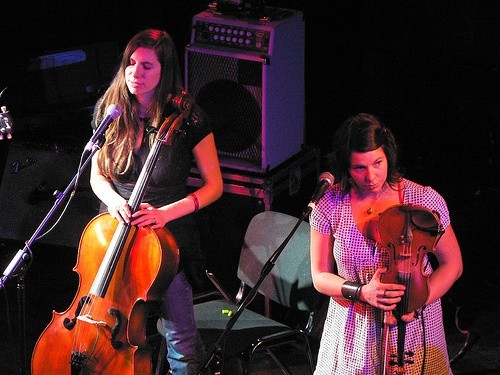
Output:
[354,189,388,214]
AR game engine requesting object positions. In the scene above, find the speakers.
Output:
[184,5,306,173]
[0,141,101,258]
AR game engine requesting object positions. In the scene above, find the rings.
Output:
[154,218,156,223]
[382,290,387,298]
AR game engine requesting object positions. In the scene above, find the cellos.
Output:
[30,90,195,375]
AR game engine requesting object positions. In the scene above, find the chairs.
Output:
[157,210,314,375]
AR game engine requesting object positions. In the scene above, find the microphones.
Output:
[32,181,50,202]
[83,104,121,152]
[305,171,335,216]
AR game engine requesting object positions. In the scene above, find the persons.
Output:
[311,113,462,375]
[89,29,223,375]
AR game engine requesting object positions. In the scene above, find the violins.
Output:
[376,202,443,375]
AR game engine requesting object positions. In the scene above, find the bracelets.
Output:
[341,280,362,299]
[186,193,199,213]
